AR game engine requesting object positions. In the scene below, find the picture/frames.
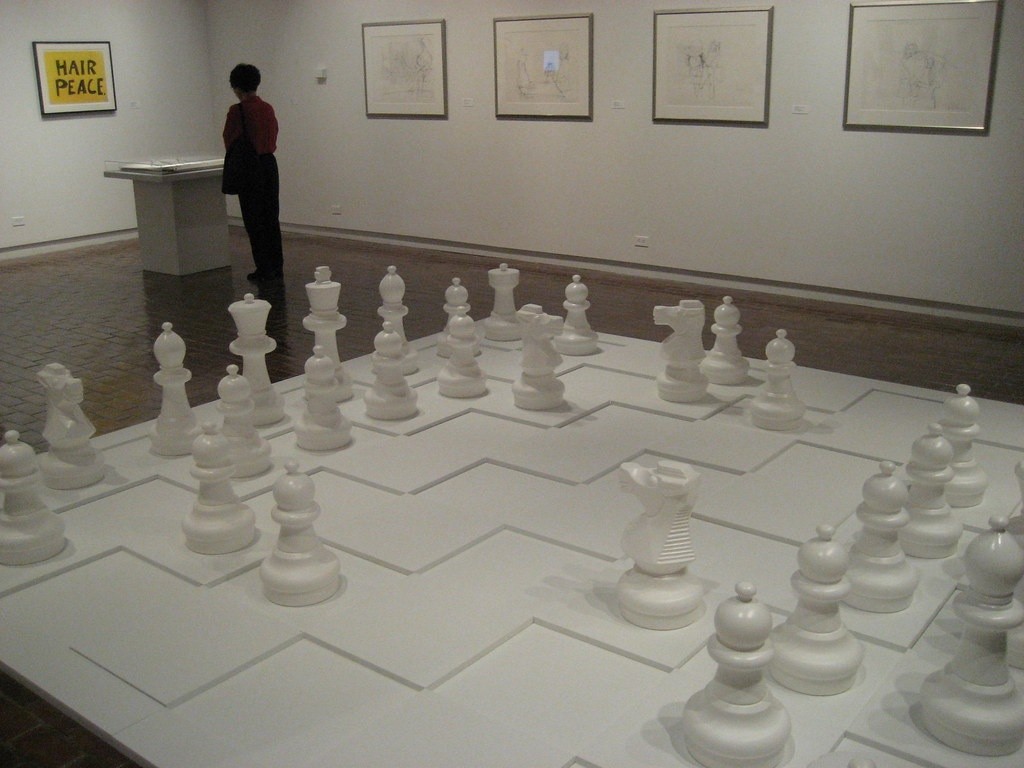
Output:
[492,12,594,122]
[842,0,1003,136]
[32,40,117,115]
[361,19,449,120]
[653,5,774,128]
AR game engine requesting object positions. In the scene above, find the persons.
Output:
[221,63,285,280]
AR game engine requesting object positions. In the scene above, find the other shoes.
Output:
[271,262,283,275]
[247,267,271,282]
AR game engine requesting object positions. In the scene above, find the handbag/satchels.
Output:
[222,103,263,194]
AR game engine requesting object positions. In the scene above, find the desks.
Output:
[105,160,237,275]
[0,321,1024,768]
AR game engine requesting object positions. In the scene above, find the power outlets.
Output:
[634,236,649,247]
[12,217,26,227]
[331,205,342,214]
